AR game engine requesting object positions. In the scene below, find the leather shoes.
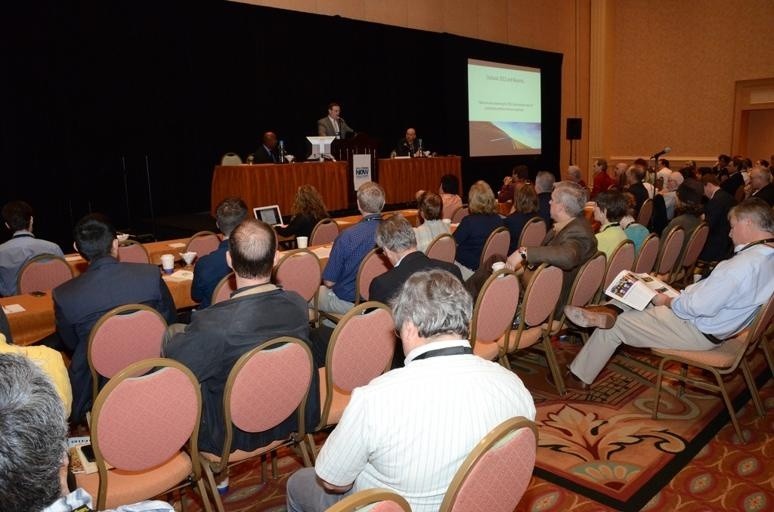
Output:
[562,304,619,330]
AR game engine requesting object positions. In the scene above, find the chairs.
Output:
[310,219,342,247]
[183,230,225,266]
[650,296,772,445]
[554,223,709,350]
[322,416,538,511]
[200,247,396,511]
[18,236,148,294]
[422,205,563,397]
[58,302,209,511]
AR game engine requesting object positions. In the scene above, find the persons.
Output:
[253,132,288,164]
[389,127,429,158]
[318,104,359,142]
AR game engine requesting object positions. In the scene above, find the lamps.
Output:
[565,117,583,165]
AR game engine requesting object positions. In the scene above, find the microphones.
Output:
[275,140,285,163]
[419,139,423,157]
[651,147,671,159]
[336,116,344,122]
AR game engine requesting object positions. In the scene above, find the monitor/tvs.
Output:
[305,135,340,162]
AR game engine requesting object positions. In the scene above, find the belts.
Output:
[701,331,724,345]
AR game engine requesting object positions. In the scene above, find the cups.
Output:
[296,236,308,249]
[694,274,702,283]
[160,253,175,274]
[491,261,506,280]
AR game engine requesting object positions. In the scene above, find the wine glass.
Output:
[178,251,197,272]
[285,155,294,164]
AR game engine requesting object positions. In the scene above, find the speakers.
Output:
[566,118,581,140]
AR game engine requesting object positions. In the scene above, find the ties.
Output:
[409,144,413,154]
[333,119,338,135]
[270,150,277,163]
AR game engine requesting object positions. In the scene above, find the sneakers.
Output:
[544,365,592,394]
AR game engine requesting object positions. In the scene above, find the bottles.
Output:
[246,155,255,166]
[278,140,286,164]
[418,139,424,157]
[408,142,414,158]
[391,150,396,159]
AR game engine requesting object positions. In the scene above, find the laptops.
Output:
[253,205,288,228]
